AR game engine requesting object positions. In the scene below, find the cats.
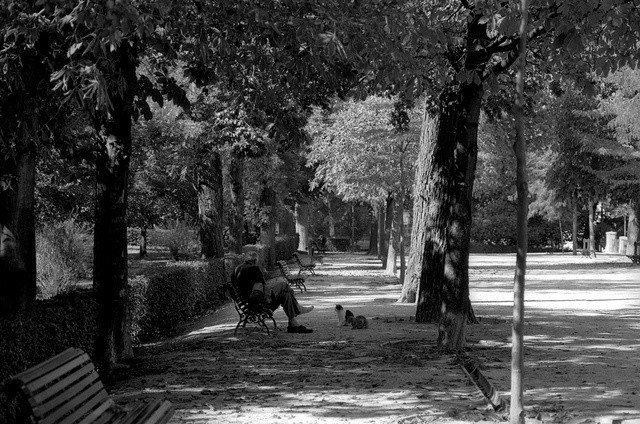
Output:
[335,303,354,326]
[347,315,368,329]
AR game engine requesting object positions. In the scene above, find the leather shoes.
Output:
[287,325,313,333]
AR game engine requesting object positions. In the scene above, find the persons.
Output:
[231,251,315,334]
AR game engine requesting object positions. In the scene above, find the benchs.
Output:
[314,242,327,255]
[227,282,277,335]
[274,260,307,294]
[292,251,316,276]
[7,346,177,424]
[310,245,324,264]
